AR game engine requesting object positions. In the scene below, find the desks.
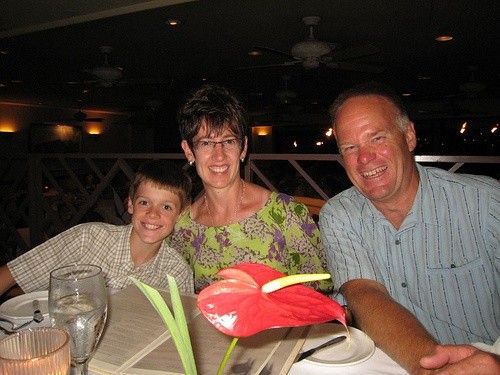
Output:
[1,286,499,375]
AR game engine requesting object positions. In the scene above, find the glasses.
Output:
[190,139,239,152]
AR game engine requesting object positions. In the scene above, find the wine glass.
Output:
[48,263,109,375]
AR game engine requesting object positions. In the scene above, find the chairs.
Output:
[289,196,326,227]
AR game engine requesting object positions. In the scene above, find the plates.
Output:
[0,290,49,319]
[299,322,377,367]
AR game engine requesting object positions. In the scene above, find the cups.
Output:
[0,327,72,375]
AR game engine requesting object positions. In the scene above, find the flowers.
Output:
[127,262,350,375]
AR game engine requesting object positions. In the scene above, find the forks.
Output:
[0,318,36,329]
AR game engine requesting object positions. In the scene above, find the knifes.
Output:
[293,334,347,364]
